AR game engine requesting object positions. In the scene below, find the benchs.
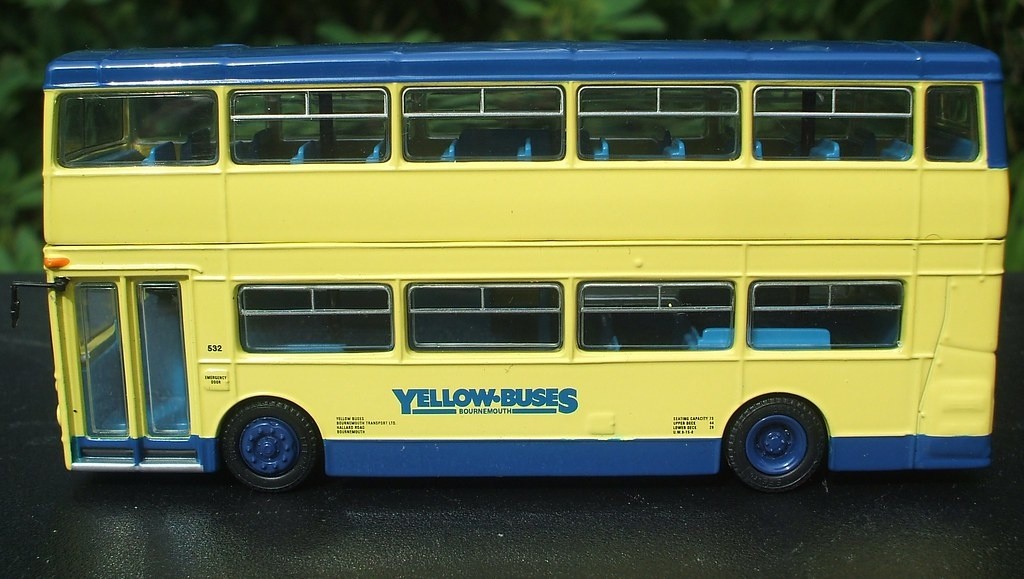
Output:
[683,327,831,349]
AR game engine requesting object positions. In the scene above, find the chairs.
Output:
[142,127,980,162]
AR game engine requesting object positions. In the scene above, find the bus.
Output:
[7,40,1011,495]
